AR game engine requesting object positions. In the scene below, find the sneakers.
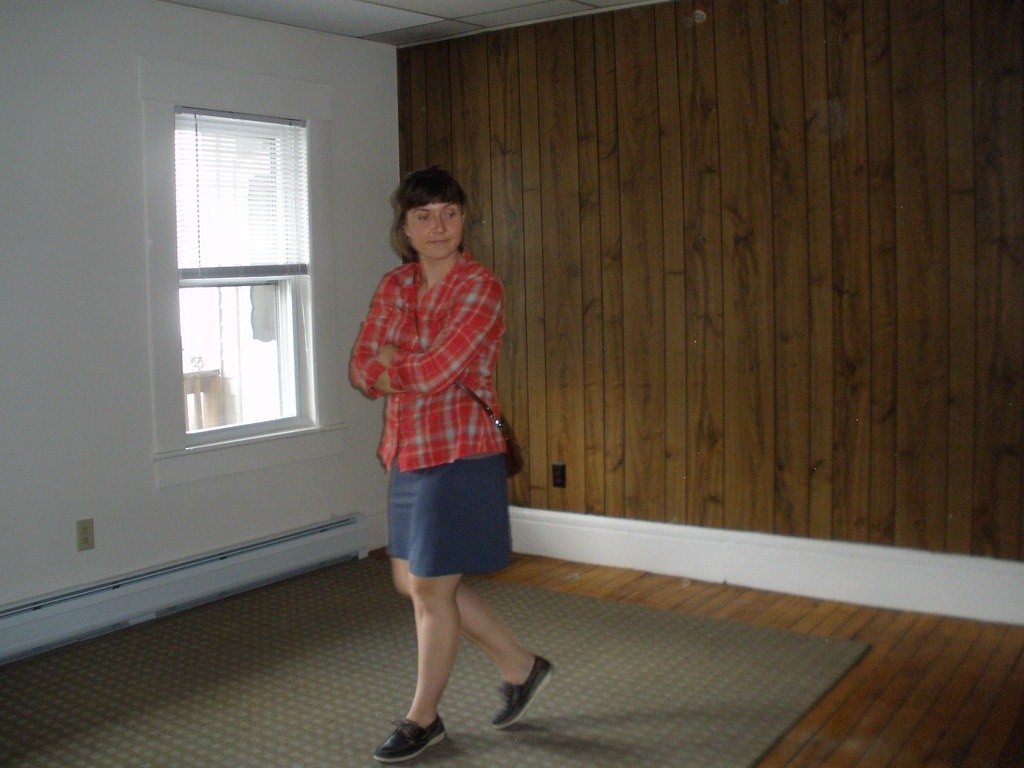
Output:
[373,714,446,762]
[490,654,556,729]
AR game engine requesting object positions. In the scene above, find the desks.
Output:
[184,368,223,431]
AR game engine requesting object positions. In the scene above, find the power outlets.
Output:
[552,463,566,488]
[76,518,95,552]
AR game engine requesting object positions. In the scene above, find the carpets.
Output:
[0,553,872,768]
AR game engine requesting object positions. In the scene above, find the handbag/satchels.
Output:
[496,417,524,476]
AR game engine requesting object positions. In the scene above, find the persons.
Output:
[347,167,554,763]
